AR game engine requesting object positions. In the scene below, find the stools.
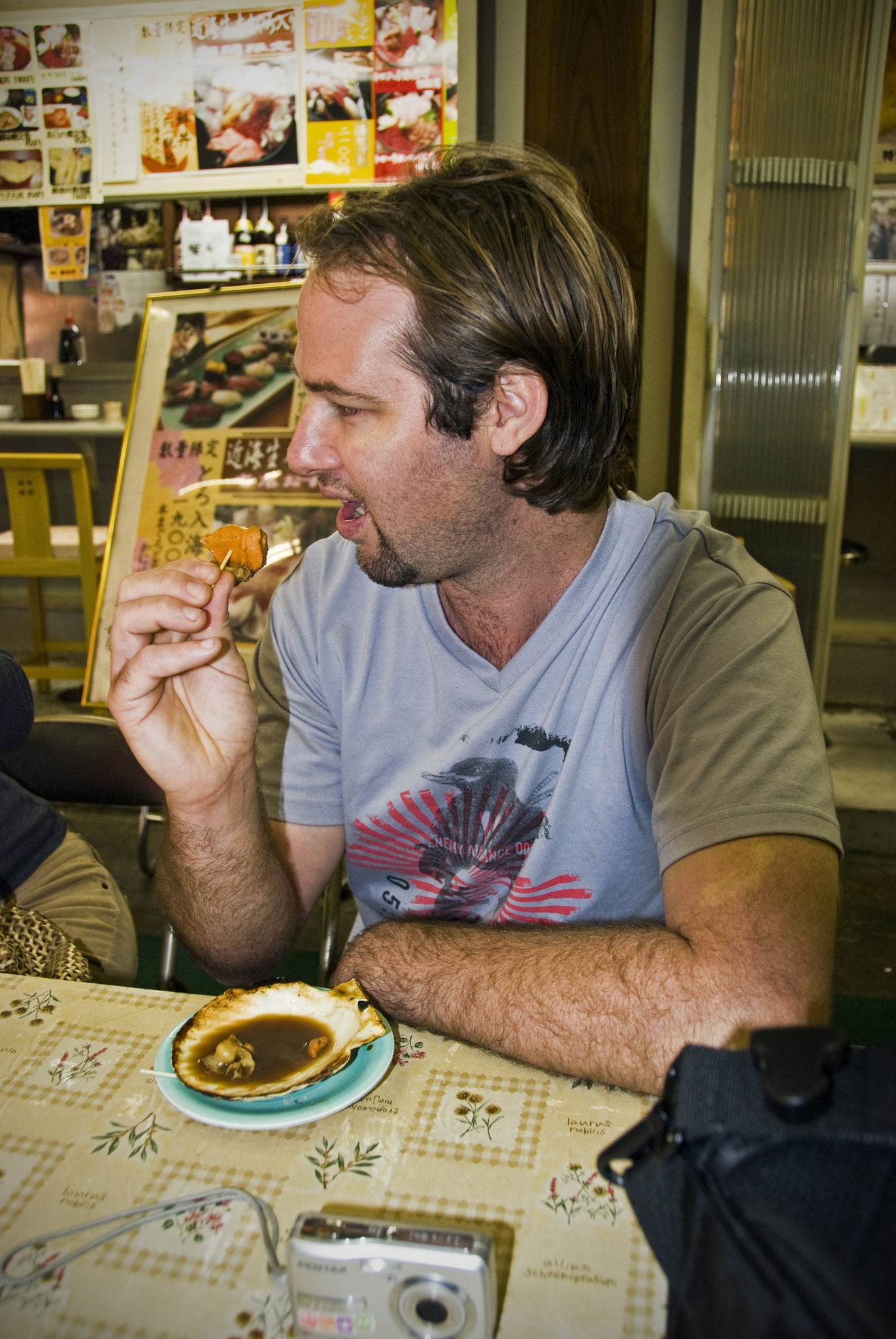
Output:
[0,454,108,692]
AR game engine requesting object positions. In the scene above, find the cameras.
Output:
[283,1211,499,1338]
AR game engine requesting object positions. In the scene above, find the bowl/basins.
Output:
[0,106,23,131]
[376,92,440,153]
[373,0,440,68]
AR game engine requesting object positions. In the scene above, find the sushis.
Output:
[163,321,297,427]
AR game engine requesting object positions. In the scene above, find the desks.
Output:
[3,970,666,1338]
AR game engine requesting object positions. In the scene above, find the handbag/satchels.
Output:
[597,1024,896,1339]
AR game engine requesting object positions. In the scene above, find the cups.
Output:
[105,400,122,420]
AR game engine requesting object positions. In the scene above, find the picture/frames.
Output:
[80,281,344,709]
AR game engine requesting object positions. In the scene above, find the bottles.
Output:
[58,316,85,365]
[171,197,307,277]
[47,373,64,420]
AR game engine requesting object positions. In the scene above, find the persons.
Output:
[0,767,139,986]
[105,139,844,1100]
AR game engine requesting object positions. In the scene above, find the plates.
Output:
[70,404,100,420]
[0,404,14,419]
[151,984,395,1131]
[161,307,298,429]
[205,122,293,164]
